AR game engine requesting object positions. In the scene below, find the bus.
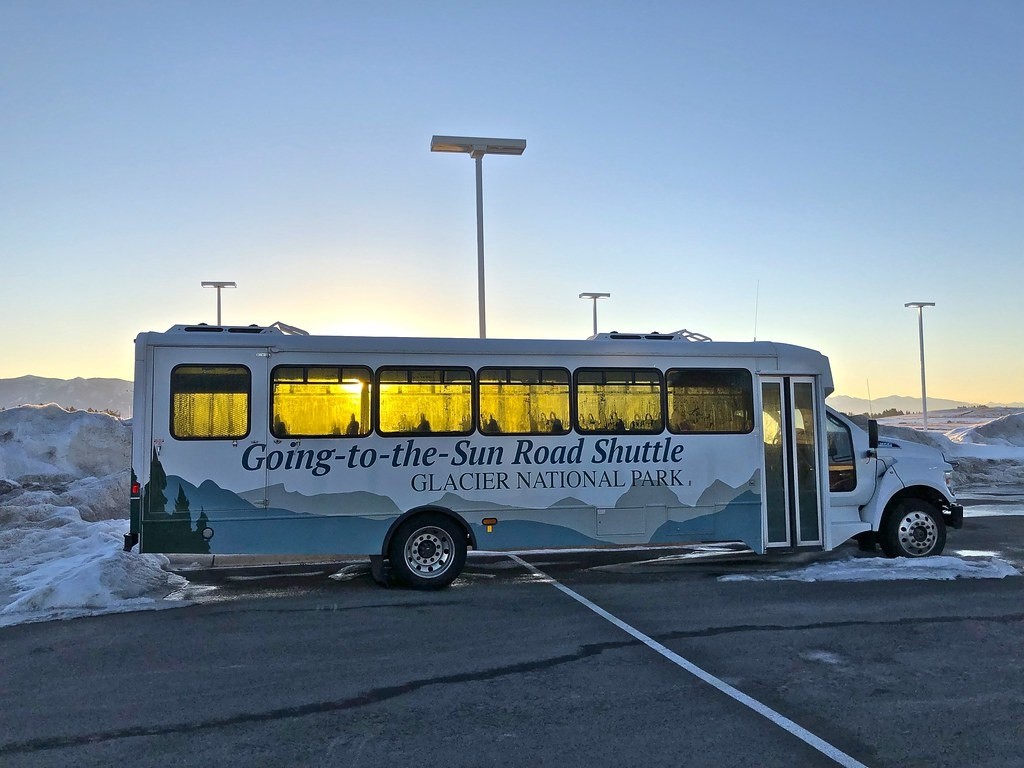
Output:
[122,330,964,591]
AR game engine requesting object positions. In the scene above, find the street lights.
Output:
[579,292,611,335]
[430,135,527,338]
[904,302,936,431]
[201,281,237,326]
